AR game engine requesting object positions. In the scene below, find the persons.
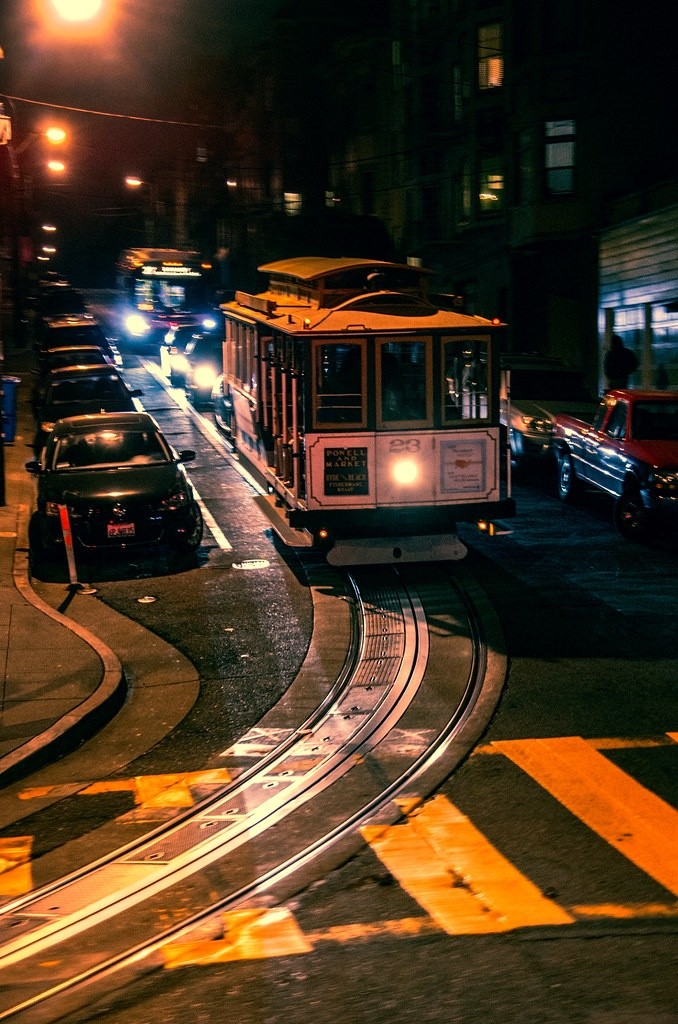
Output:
[603,334,640,394]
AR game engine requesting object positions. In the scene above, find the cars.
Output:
[182,329,224,406]
[159,323,212,384]
[212,370,236,434]
[34,362,145,437]
[19,410,202,560]
[37,345,117,400]
[24,276,116,360]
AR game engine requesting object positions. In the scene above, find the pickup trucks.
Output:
[553,388,678,546]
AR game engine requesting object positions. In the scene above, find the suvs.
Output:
[464,357,599,466]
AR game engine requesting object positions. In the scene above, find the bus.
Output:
[113,247,207,345]
[217,254,514,566]
